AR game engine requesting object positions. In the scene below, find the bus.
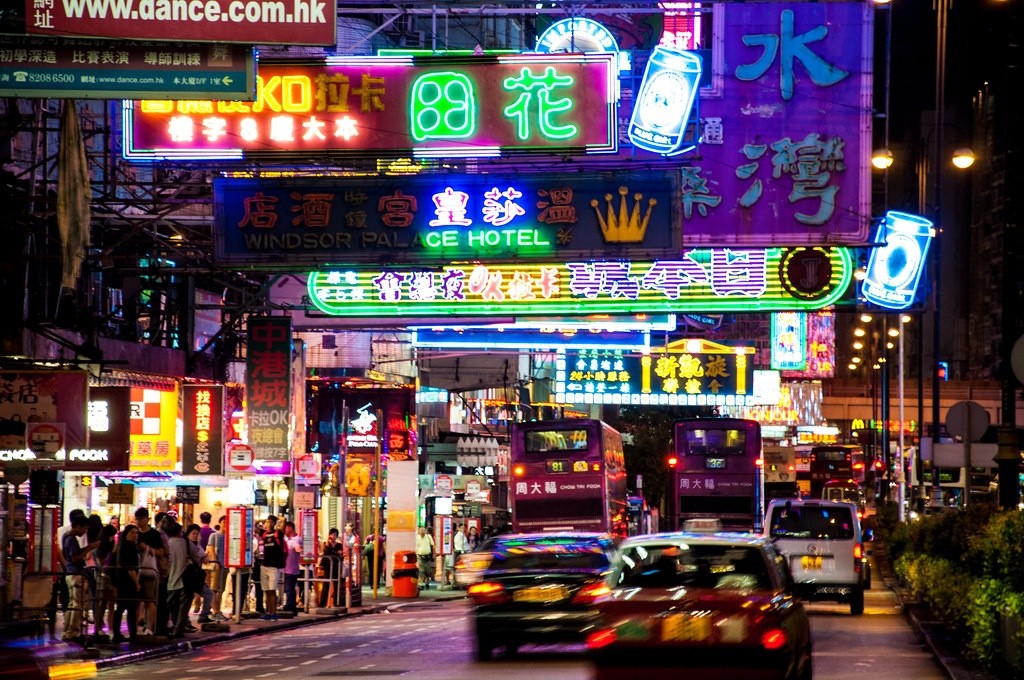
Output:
[764,445,797,483]
[510,418,627,536]
[794,445,865,483]
[657,418,762,532]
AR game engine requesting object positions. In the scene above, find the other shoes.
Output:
[270,614,278,621]
[422,578,425,583]
[424,583,430,589]
[377,586,380,588]
[446,582,451,585]
[193,607,200,614]
[65,618,197,646]
[371,587,373,589]
[255,607,266,614]
[197,615,214,624]
[259,614,270,620]
[431,578,435,581]
[215,612,227,622]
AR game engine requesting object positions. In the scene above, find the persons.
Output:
[39,507,230,642]
[342,524,360,577]
[363,527,386,589]
[417,527,435,589]
[445,523,479,584]
[231,515,303,622]
[319,528,344,608]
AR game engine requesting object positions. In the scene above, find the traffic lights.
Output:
[874,460,883,477]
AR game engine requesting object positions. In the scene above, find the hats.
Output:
[167,510,178,520]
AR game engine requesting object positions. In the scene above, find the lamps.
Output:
[25,318,103,378]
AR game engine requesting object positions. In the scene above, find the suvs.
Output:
[763,499,874,615]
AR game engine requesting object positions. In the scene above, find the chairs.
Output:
[658,555,677,575]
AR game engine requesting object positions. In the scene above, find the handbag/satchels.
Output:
[201,533,217,571]
[362,535,374,555]
[180,563,206,593]
[139,545,158,577]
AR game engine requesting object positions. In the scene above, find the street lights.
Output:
[848,312,912,524]
[871,148,978,519]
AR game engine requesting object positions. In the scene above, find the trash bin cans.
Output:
[390,550,420,599]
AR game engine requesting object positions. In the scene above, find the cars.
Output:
[864,550,874,590]
[454,530,619,661]
[587,532,813,680]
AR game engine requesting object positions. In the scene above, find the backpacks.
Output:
[262,529,288,554]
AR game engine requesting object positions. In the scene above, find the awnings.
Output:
[482,505,508,515]
